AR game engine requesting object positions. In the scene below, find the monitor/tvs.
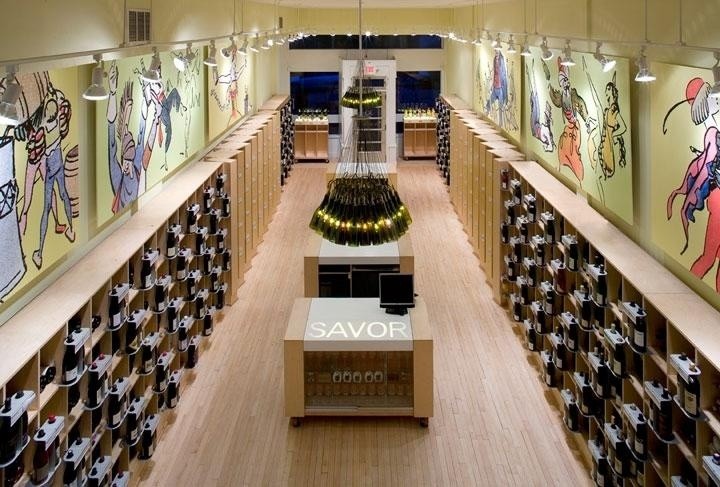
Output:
[378,271,415,315]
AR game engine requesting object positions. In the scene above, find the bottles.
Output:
[280,100,293,185]
[500,170,720,486]
[1,172,233,486]
[297,108,327,121]
[306,352,414,397]
[309,176,412,247]
[435,98,450,189]
[340,87,383,109]
[403,101,434,117]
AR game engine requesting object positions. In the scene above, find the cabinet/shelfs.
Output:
[204,96,293,308]
[449,109,525,307]
[501,158,719,486]
[437,91,472,189]
[403,117,437,161]
[293,117,329,163]
[2,161,230,487]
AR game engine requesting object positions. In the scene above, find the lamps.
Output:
[304,37,412,248]
[490,34,656,86]
[1,35,250,128]
